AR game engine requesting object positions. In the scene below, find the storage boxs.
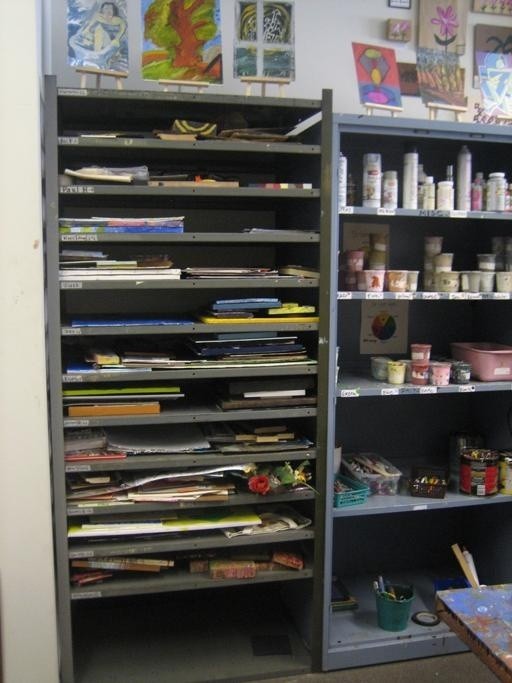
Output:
[450,341,512,382]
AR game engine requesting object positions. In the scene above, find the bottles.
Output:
[340,142,512,211]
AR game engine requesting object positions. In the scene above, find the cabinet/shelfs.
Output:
[43,72,334,683]
[323,112,512,671]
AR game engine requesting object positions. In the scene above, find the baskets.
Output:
[410,466,449,499]
[334,472,369,508]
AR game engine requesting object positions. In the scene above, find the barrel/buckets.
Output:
[375,584,416,631]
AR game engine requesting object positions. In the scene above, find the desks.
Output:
[434,582,512,682]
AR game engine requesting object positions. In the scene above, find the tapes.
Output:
[412,611,440,626]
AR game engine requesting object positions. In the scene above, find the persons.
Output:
[82,2,126,50]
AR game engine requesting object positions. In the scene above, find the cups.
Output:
[345,232,512,291]
[373,342,472,384]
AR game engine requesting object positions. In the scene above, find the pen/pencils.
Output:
[373,576,395,598]
